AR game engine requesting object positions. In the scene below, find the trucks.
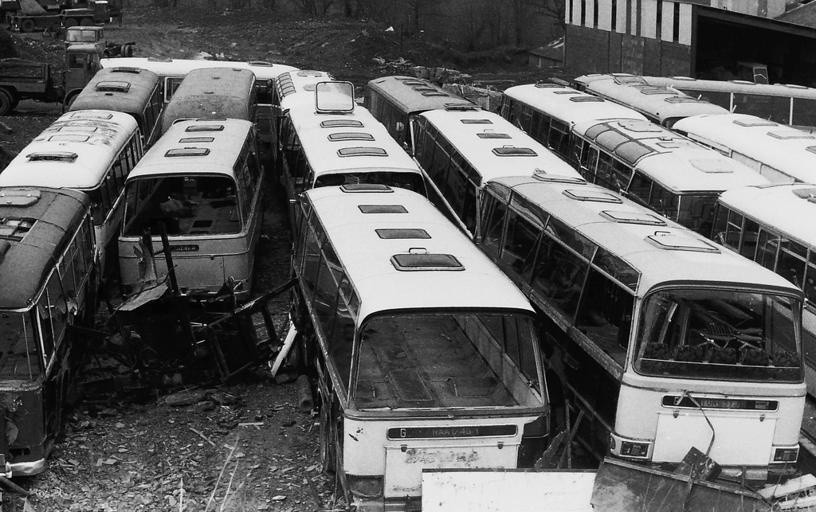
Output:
[0,0,136,116]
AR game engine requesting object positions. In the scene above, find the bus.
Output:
[709,180,816,405]
[472,170,807,501]
[0,180,102,478]
[285,183,556,512]
